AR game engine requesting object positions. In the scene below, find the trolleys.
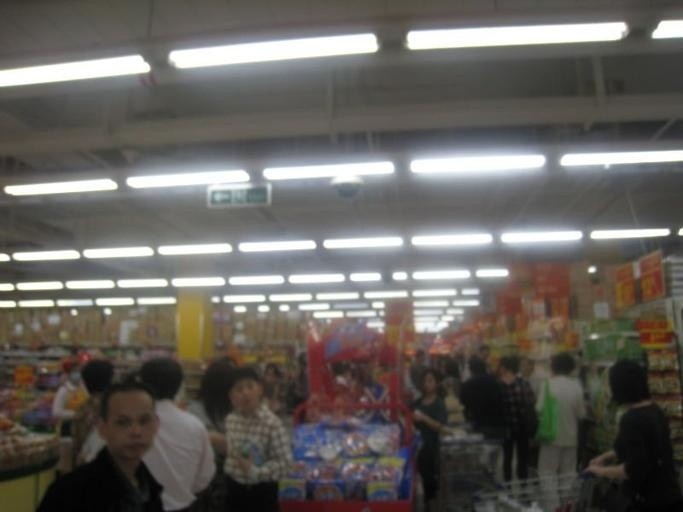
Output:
[439,430,593,512]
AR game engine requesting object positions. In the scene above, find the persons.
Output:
[30,323,682,509]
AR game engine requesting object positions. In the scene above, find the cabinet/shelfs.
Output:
[584,331,644,421]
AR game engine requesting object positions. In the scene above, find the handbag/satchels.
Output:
[534,378,559,444]
